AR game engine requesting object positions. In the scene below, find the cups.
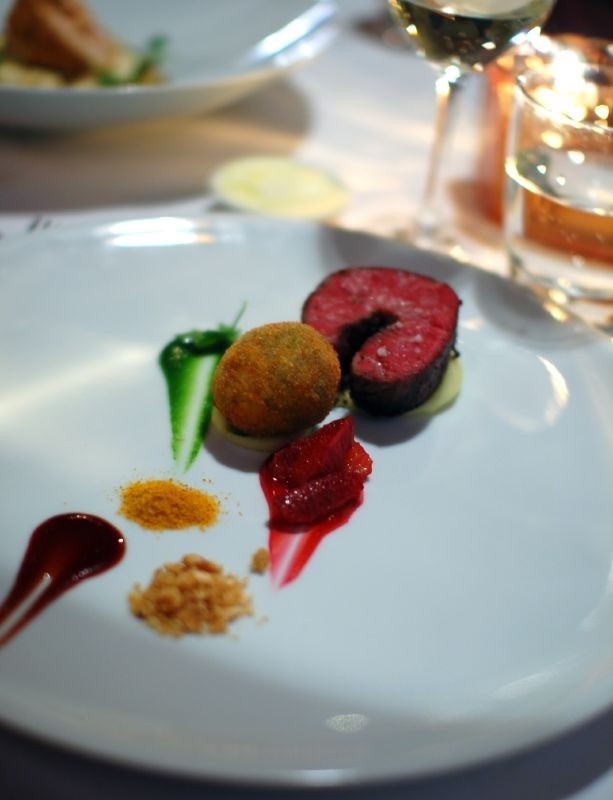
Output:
[502,34,613,330]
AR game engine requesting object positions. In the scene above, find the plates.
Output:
[0,0,365,132]
[208,154,351,223]
[0,200,612,787]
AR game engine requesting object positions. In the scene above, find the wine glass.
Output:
[388,0,555,279]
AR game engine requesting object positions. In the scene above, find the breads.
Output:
[0,0,138,82]
